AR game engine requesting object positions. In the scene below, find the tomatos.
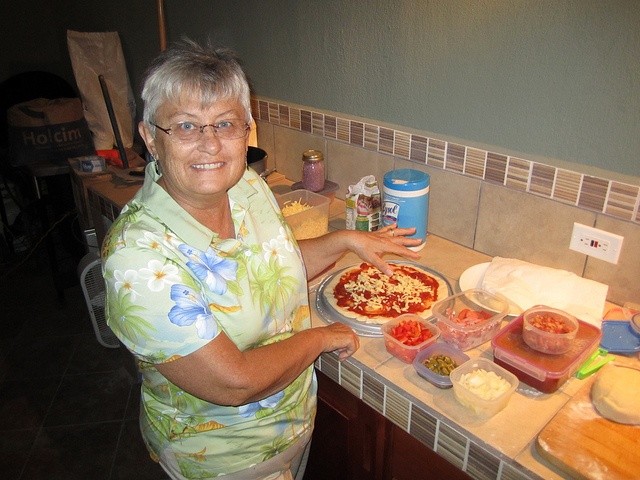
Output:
[384,320,435,363]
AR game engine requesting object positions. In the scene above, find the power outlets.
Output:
[568,222,624,265]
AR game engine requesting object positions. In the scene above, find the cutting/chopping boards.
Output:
[535,363,640,480]
[314,259,455,338]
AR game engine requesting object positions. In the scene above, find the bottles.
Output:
[301,149,325,192]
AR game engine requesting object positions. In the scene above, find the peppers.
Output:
[418,352,461,388]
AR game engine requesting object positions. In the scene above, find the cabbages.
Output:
[455,368,512,417]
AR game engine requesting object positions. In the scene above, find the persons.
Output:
[100,34,423,480]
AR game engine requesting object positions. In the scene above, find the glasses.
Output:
[148,116,252,141]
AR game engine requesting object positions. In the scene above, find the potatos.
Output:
[280,197,329,240]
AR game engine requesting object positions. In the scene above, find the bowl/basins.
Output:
[490,301,602,393]
[276,189,331,239]
[450,358,520,421]
[413,342,470,388]
[522,305,581,355]
[431,289,512,352]
[380,313,441,364]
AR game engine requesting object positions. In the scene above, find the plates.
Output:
[458,262,523,317]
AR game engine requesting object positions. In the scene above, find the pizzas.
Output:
[321,264,448,326]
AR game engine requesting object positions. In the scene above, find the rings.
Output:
[390,228,395,238]
